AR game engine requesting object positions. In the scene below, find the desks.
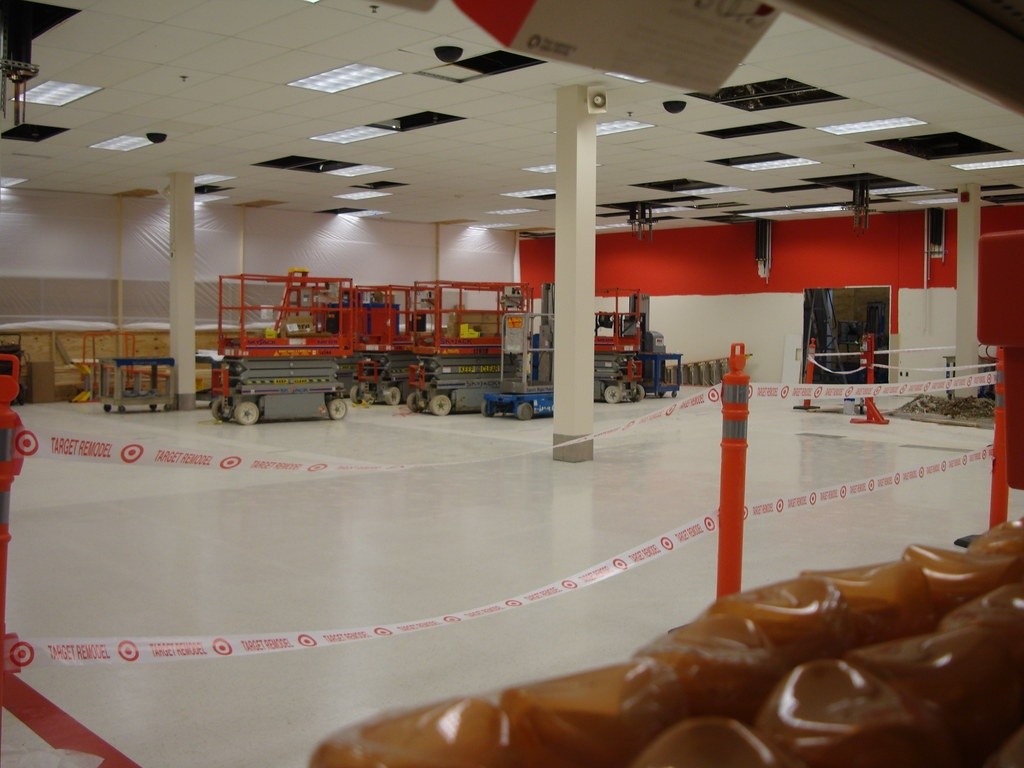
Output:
[639,352,684,397]
[99,357,176,412]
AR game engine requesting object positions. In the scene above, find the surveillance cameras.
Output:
[663,100,686,114]
[434,46,463,62]
[147,132,167,143]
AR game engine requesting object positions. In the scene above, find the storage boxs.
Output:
[258,327,278,339]
[446,312,504,338]
[287,314,316,336]
[453,0,783,96]
[27,361,56,404]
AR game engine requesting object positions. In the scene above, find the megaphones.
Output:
[587,85,608,114]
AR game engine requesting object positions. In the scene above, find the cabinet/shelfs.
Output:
[501,312,554,394]
